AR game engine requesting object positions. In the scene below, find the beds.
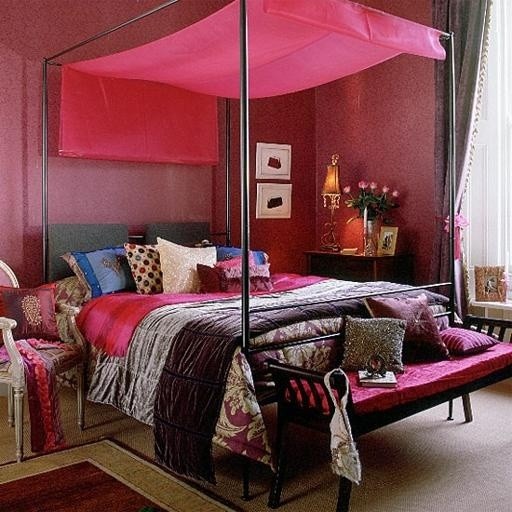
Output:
[42,0,455,501]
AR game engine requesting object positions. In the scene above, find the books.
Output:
[342,249,355,255]
[357,369,398,388]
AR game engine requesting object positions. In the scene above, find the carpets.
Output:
[0,438,248,512]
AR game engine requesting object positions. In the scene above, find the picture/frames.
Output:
[474,265,506,303]
[378,226,399,256]
[255,183,293,219]
[255,142,292,182]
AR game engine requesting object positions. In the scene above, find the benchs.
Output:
[263,314,511,511]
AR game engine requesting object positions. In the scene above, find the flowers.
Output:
[343,180,401,225]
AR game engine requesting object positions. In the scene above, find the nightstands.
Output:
[304,249,416,286]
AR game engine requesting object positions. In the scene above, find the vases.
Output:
[364,221,377,256]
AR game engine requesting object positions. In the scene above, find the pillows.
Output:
[218,246,269,265]
[60,246,134,299]
[124,242,162,294]
[440,325,500,352]
[0,285,61,342]
[362,294,451,362]
[197,263,220,293]
[340,314,407,374]
[218,264,273,292]
[156,236,217,295]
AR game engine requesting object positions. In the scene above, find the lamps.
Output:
[320,152,342,252]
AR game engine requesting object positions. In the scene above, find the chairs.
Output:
[1,260,89,464]
